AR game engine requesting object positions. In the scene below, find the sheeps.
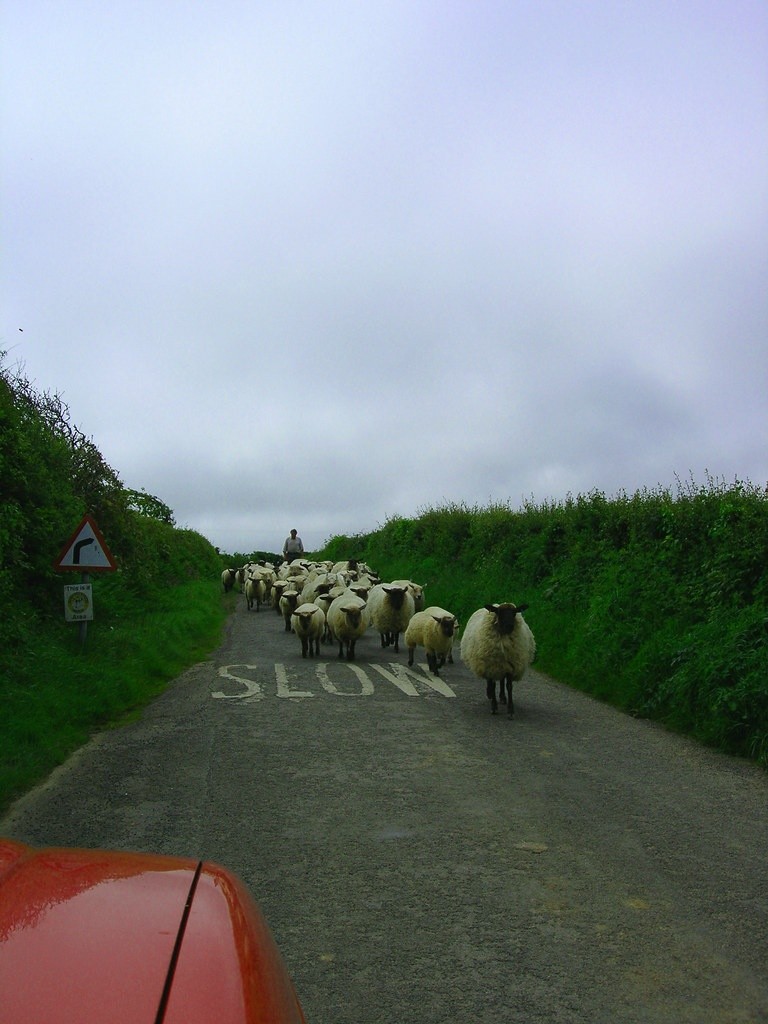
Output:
[221,558,429,661]
[458,601,538,721]
[403,605,460,678]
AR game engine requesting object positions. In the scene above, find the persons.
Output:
[282,529,303,565]
